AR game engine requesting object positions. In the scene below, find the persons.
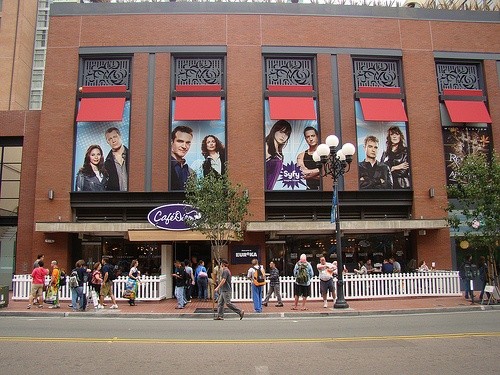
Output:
[247,259,265,313]
[262,260,284,307]
[201,135,225,181]
[364,258,376,278]
[211,258,223,303]
[459,253,478,301]
[380,126,411,189]
[88,261,108,306]
[316,256,338,308]
[265,120,293,190]
[75,144,109,191]
[103,127,128,191]
[127,260,143,306]
[478,256,493,301]
[290,254,314,311]
[214,258,245,320]
[94,257,118,309]
[170,125,197,190]
[357,135,393,189]
[180,254,211,302]
[68,260,91,312]
[26,254,49,309]
[48,260,61,309]
[353,261,367,278]
[297,126,321,190]
[170,259,187,309]
[381,256,401,295]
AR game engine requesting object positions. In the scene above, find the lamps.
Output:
[429,187,435,198]
[48,190,54,200]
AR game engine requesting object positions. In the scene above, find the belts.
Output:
[320,278,333,282]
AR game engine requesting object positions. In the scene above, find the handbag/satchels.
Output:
[198,267,208,283]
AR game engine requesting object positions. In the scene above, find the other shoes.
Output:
[301,307,308,310]
[254,310,262,312]
[36,305,44,309]
[47,305,57,309]
[187,300,191,303]
[128,300,135,305]
[262,302,268,306]
[68,304,73,307]
[276,303,283,307]
[26,304,31,308]
[214,316,224,320]
[56,304,60,307]
[102,302,107,306]
[290,306,298,310]
[95,304,105,309]
[239,310,244,320]
[109,305,118,308]
[324,303,327,308]
[175,306,183,309]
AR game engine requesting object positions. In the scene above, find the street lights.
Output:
[313,135,356,310]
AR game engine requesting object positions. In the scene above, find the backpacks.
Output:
[58,270,66,286]
[251,266,265,286]
[68,268,80,288]
[295,262,308,283]
